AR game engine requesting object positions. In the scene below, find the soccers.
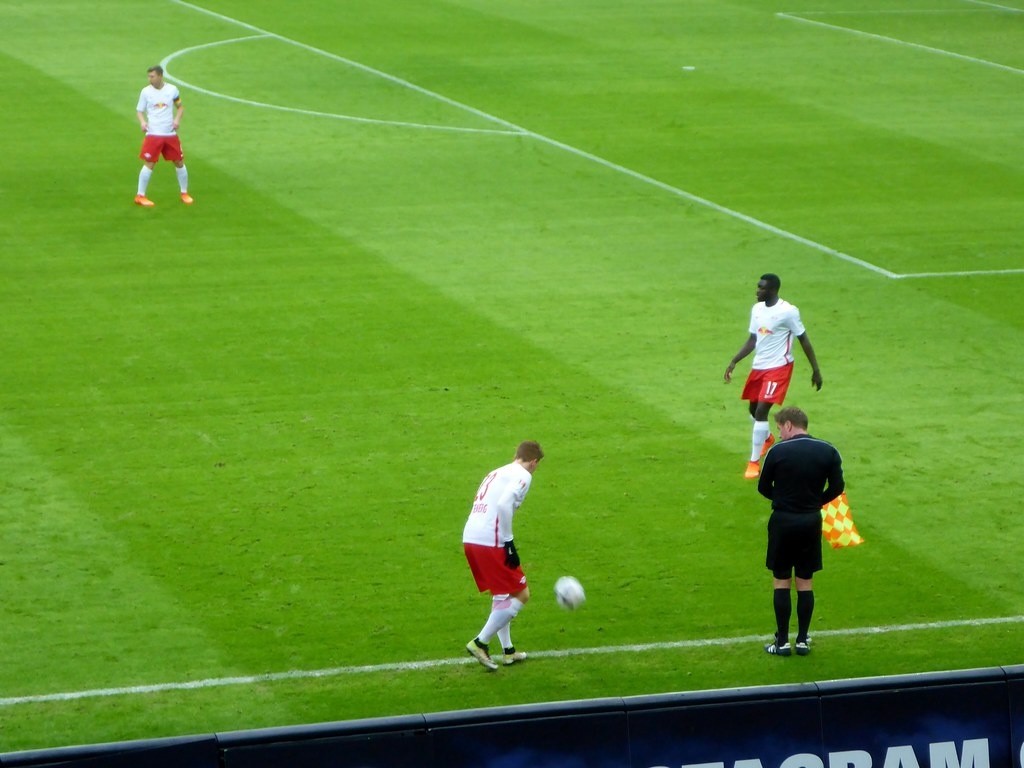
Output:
[555,575,585,610]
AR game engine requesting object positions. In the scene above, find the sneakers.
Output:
[465,638,499,670]
[759,433,775,456]
[134,194,154,205]
[764,642,792,656]
[503,652,526,665]
[181,193,193,204]
[744,461,760,479]
[796,644,810,655]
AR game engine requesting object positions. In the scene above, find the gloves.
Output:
[504,540,520,569]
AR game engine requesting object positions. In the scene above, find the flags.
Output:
[821,492,865,549]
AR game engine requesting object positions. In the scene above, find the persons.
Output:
[463,440,544,669]
[758,406,845,658]
[134,65,194,206]
[725,274,823,478]
